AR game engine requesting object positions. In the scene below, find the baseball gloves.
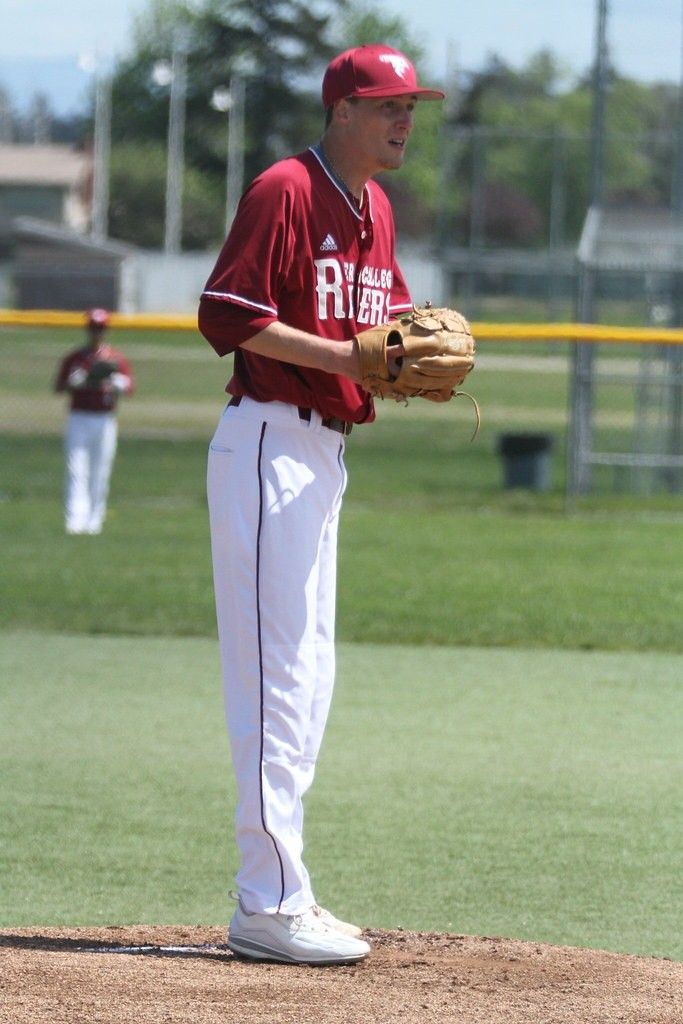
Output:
[353,300,475,404]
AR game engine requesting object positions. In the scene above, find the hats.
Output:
[84,307,110,329]
[321,44,446,111]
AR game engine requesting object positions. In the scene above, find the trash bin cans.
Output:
[493,431,554,492]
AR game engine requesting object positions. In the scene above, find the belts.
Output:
[223,393,355,437]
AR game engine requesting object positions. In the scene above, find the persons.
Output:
[198,44,475,968]
[54,307,134,538]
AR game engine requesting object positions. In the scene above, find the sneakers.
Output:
[226,889,371,967]
